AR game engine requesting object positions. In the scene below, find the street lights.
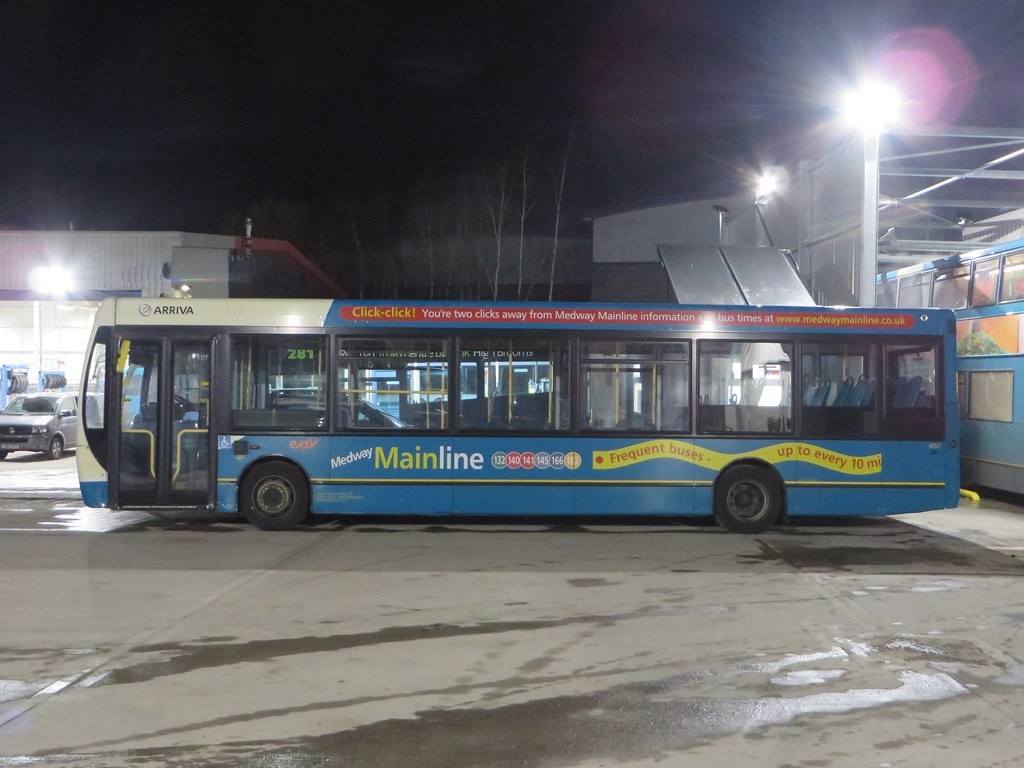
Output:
[752,170,782,245]
[844,77,904,309]
[28,260,79,393]
[714,203,729,247]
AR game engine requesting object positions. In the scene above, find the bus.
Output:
[71,285,967,536]
[877,239,1024,494]
[125,359,550,429]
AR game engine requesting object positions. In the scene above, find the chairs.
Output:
[797,373,924,407]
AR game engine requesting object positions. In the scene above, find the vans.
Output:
[0,390,103,461]
